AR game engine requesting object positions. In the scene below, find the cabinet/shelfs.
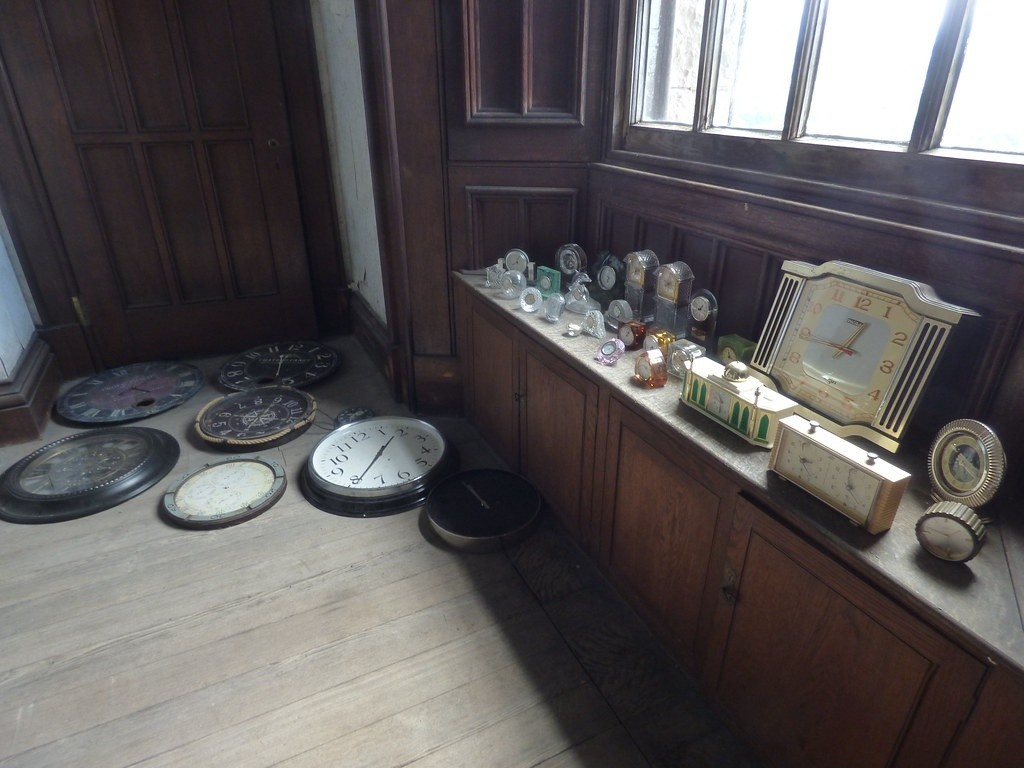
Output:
[451,268,645,557]
[592,372,1024,767]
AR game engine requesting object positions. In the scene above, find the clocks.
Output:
[161,454,287,531]
[916,499,987,566]
[1,425,181,525]
[216,339,345,394]
[926,417,1009,524]
[750,259,985,456]
[317,405,378,431]
[768,412,913,537]
[193,383,319,453]
[679,356,798,450]
[54,360,206,426]
[425,466,543,552]
[297,415,461,519]
[483,242,760,391]
